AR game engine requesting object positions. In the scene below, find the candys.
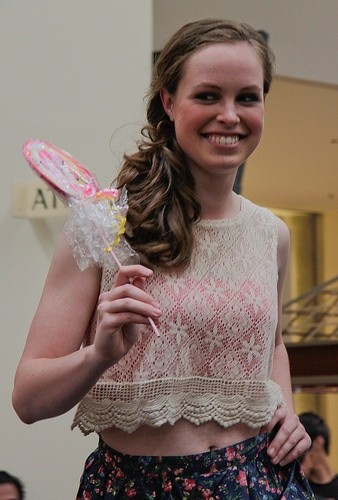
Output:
[21,139,101,202]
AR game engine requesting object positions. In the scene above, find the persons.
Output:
[298,412,338,500]
[11,19,315,500]
